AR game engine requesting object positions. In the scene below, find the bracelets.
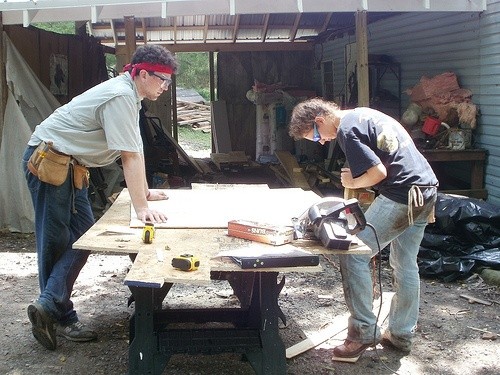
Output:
[147,192,150,198]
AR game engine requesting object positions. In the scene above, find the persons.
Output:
[288,98,439,359]
[23,44,178,352]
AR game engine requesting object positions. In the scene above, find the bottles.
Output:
[422,117,441,136]
[448,129,466,150]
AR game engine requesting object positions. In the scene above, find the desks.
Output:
[424,149,489,200]
[72,188,371,375]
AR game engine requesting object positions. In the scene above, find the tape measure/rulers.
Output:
[171,253,200,271]
[142,225,155,243]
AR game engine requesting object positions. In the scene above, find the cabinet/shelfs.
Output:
[367,61,403,121]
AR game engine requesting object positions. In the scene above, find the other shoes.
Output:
[333,337,381,358]
[381,328,412,353]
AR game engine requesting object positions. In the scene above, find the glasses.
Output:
[312,122,321,142]
[151,73,171,89]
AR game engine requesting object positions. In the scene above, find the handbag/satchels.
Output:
[73,164,91,190]
[26,141,71,186]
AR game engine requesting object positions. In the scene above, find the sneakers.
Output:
[27,302,57,351]
[54,320,97,343]
